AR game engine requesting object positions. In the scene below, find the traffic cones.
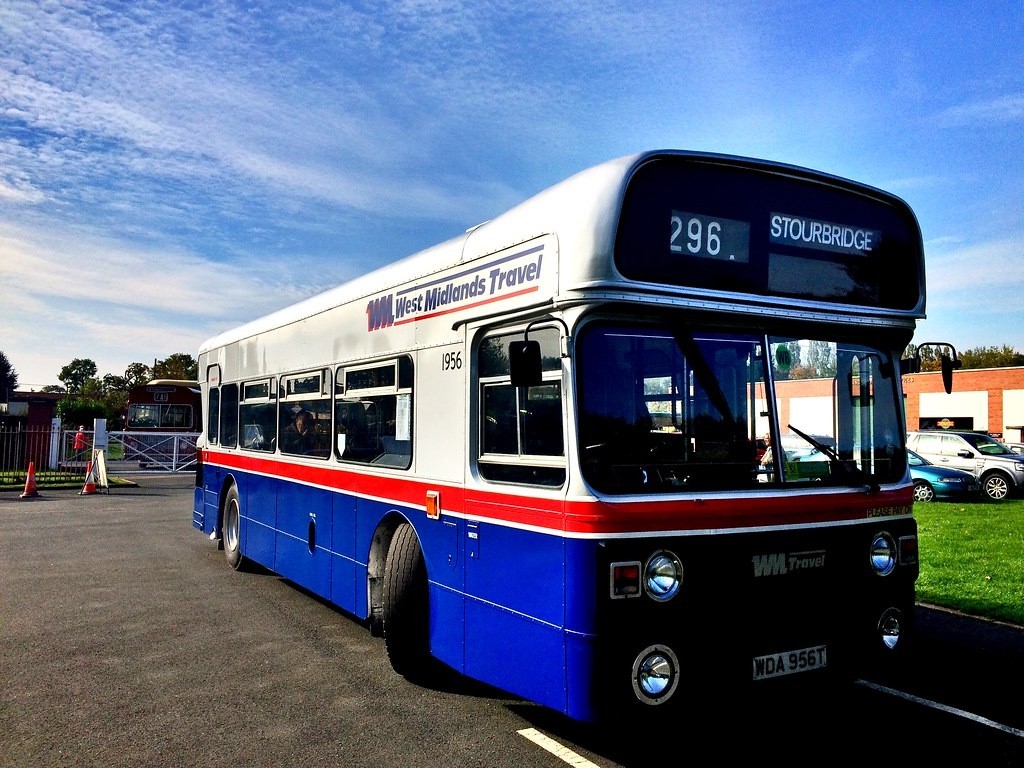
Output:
[19,461,41,498]
[78,461,97,495]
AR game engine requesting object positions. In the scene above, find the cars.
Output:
[750,433,836,484]
[799,449,974,503]
[904,428,1024,501]
[648,412,684,434]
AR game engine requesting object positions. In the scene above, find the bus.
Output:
[189,149,965,721]
[123,378,205,470]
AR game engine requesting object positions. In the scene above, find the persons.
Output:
[74,425,88,462]
[282,400,396,455]
